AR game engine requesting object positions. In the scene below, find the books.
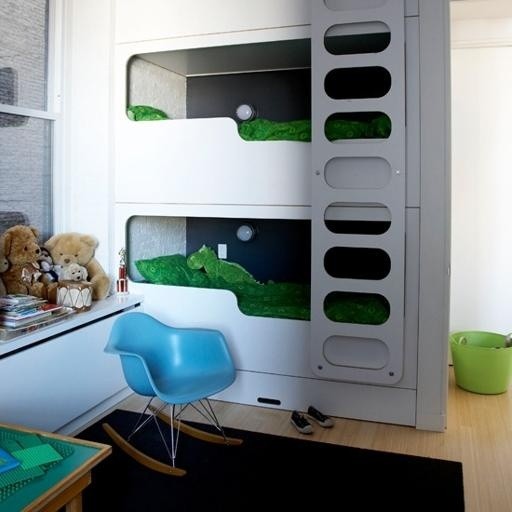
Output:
[0,294,77,343]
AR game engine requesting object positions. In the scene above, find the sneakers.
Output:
[307,406,334,428]
[290,410,314,434]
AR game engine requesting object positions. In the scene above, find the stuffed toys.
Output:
[0,224,112,304]
[187,244,267,288]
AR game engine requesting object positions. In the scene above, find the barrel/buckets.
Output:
[450,331,512,395]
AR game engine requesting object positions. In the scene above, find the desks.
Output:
[0,422,110,512]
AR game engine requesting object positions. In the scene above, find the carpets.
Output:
[75,407,465,512]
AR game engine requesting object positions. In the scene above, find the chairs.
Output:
[98,312,244,482]
